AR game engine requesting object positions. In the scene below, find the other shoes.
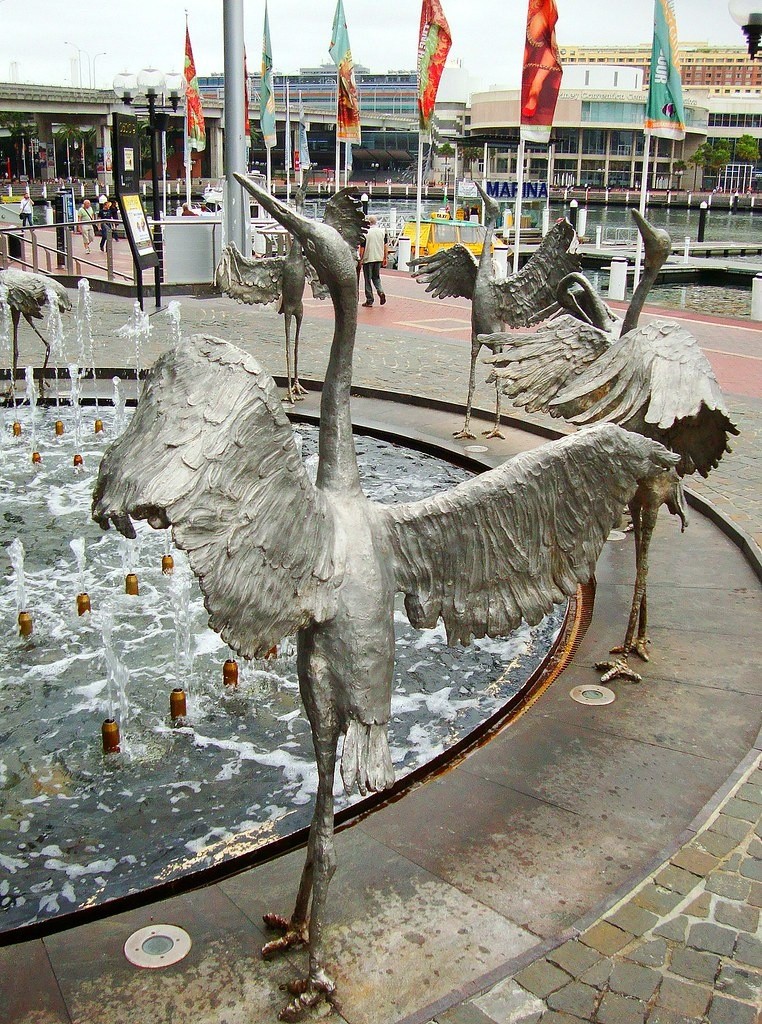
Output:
[116,239,119,242]
[100,246,104,251]
[86,247,91,254]
[362,302,372,307]
[379,292,386,305]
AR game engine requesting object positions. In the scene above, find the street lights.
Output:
[78,49,91,93]
[674,170,685,202]
[444,164,452,188]
[112,64,183,283]
[597,168,606,192]
[371,162,380,186]
[190,160,197,186]
[93,52,107,89]
[308,162,318,185]
[64,41,83,95]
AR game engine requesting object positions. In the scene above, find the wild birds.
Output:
[473,207,739,682]
[0,269,72,397]
[405,180,583,442]
[214,165,370,404]
[89,174,679,1022]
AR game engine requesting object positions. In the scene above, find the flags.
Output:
[644,0,688,141]
[520,0,563,143]
[284,94,312,170]
[327,0,364,146]
[161,131,168,171]
[21,140,86,165]
[345,143,354,171]
[244,2,277,149]
[183,17,206,152]
[182,117,193,171]
[415,0,453,144]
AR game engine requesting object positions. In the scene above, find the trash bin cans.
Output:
[7,233,24,258]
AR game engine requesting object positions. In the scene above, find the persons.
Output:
[746,186,753,195]
[4,173,87,187]
[732,186,741,193]
[716,184,723,194]
[78,200,119,254]
[356,214,387,307]
[181,203,206,216]
[20,193,34,232]
[446,202,479,221]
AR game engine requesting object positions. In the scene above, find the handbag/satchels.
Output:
[93,224,98,232]
[19,212,24,220]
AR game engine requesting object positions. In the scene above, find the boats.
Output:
[386,182,514,274]
[175,170,290,254]
